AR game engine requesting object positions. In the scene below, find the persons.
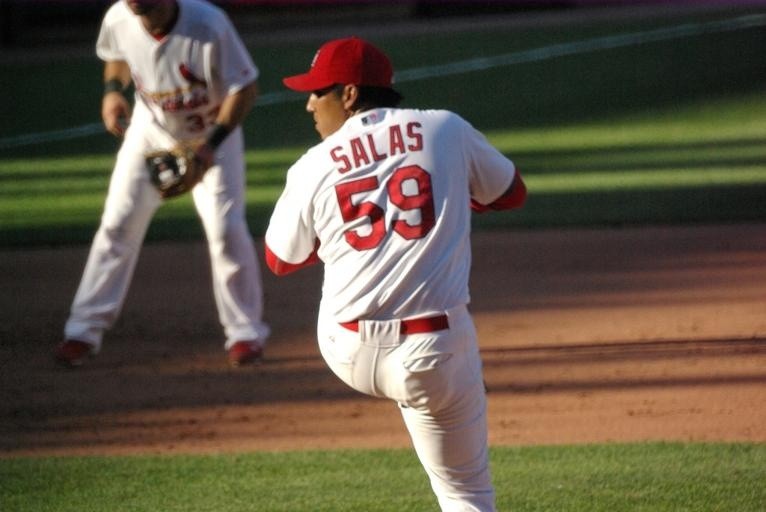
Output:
[263,37,526,512]
[55,0,271,368]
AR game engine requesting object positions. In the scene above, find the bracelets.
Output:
[103,79,123,94]
[203,123,230,148]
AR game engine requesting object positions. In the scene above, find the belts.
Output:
[338,313,449,336]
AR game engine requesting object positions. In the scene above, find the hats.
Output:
[281,35,395,93]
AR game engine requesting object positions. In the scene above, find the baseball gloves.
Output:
[145,134,214,199]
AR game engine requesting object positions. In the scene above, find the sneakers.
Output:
[53,339,95,367]
[227,339,264,367]
